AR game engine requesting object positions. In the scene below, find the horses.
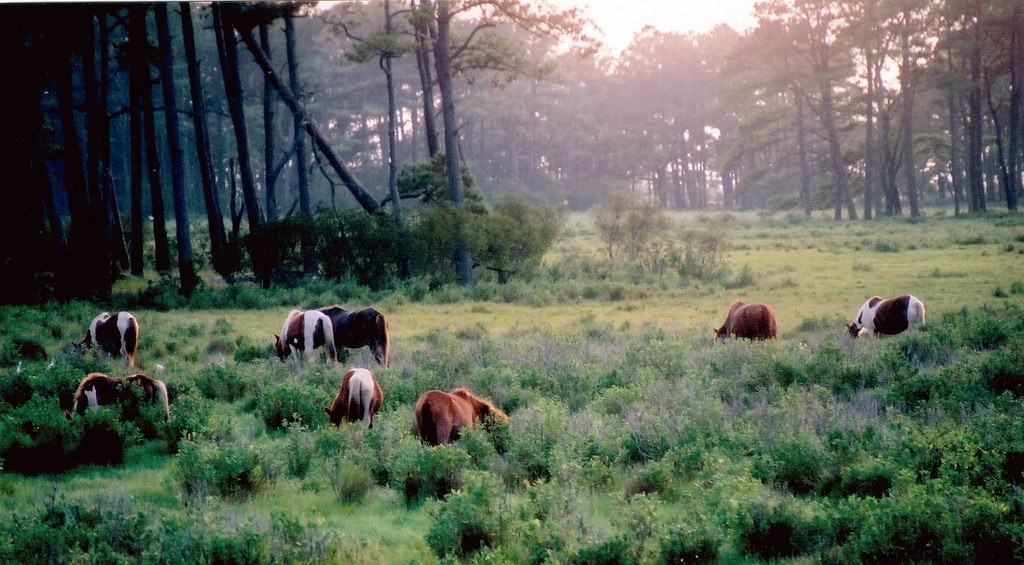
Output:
[712,302,778,344]
[69,310,139,370]
[65,371,170,424]
[273,305,388,376]
[323,366,383,433]
[415,388,512,447]
[845,296,926,341]
